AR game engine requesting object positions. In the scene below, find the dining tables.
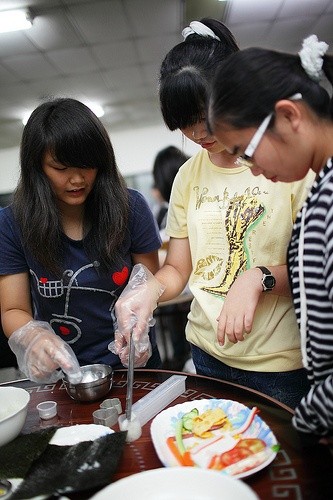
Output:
[0,368,333,500]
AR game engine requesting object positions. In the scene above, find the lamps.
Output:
[0,8,36,34]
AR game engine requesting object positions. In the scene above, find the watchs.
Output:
[257,266,276,293]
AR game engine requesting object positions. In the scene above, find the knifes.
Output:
[124,330,138,422]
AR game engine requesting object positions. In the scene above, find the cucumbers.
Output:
[182,408,198,431]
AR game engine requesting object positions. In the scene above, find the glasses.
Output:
[235,93,303,168]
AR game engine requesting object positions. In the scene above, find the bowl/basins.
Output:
[0,386,32,448]
[62,363,114,401]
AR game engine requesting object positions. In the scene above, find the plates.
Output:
[149,398,280,484]
[86,467,261,499]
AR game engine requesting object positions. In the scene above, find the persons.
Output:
[114,20,316,411]
[0,99,162,378]
[208,35,333,444]
